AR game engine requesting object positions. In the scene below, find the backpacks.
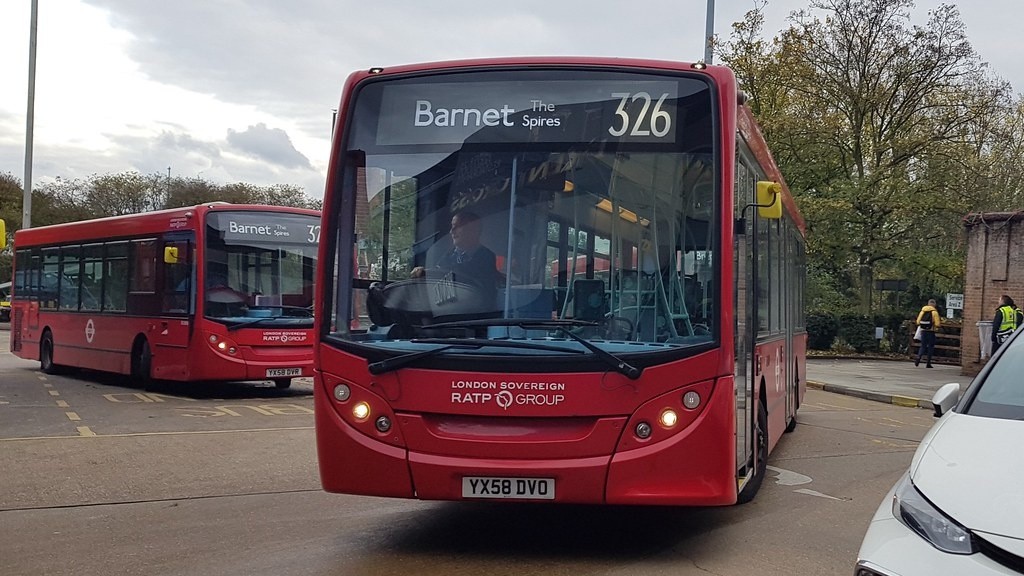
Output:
[919,310,936,331]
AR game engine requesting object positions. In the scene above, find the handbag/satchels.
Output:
[996,327,1015,345]
[912,326,922,340]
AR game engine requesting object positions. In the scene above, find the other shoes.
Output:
[915,360,919,367]
[926,365,933,368]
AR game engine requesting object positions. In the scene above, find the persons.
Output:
[991,295,1024,357]
[409,211,498,307]
[914,300,941,368]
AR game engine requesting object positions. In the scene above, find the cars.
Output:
[853,318,1023,575]
[0,281,12,323]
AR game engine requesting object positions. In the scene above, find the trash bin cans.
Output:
[976,321,994,362]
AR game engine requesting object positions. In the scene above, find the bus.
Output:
[9,201,372,394]
[315,55,807,508]
